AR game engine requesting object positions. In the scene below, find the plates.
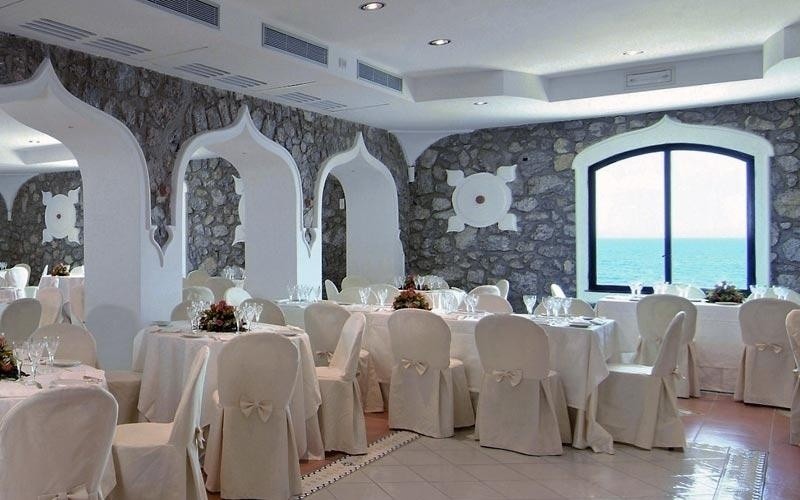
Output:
[568,322,591,327]
[53,358,81,368]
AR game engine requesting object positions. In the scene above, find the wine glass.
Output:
[398,275,442,290]
[13,335,64,385]
[749,284,789,303]
[185,298,263,335]
[541,297,552,316]
[637,281,643,297]
[561,298,572,314]
[652,282,687,300]
[287,284,320,306]
[553,297,561,315]
[463,293,479,313]
[629,281,636,297]
[522,295,536,313]
[358,287,387,306]
